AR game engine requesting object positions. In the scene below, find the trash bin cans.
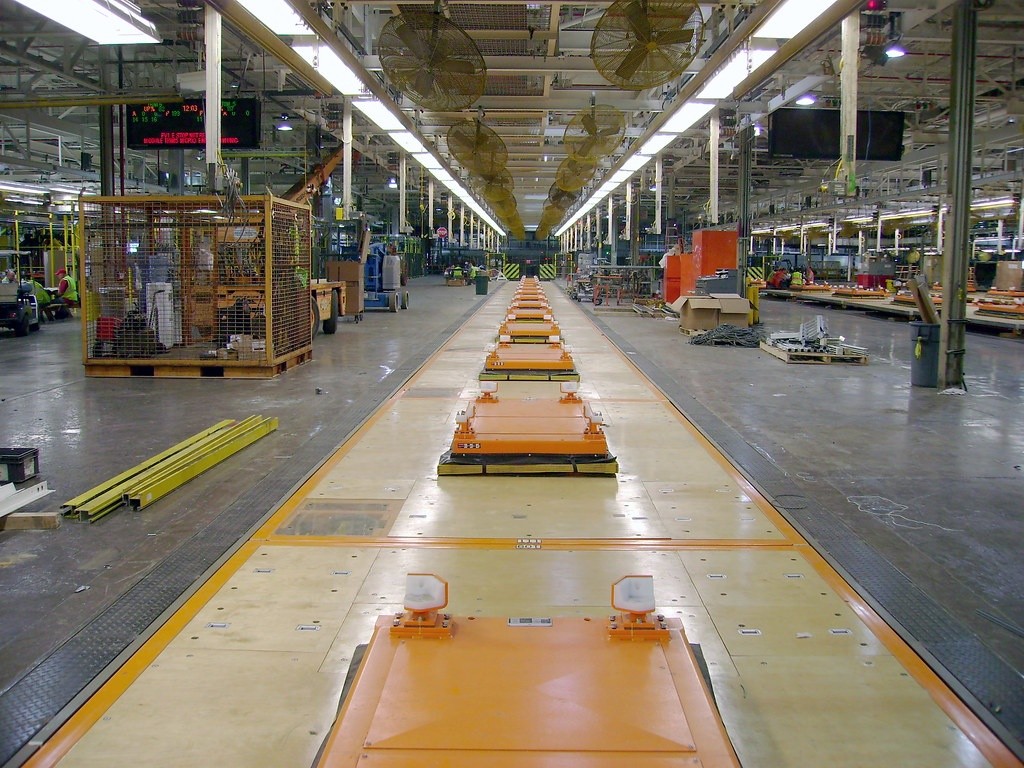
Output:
[909,320,941,387]
[473,275,489,295]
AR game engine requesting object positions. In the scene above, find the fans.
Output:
[588,0,705,91]
[446,102,626,240]
[377,0,488,114]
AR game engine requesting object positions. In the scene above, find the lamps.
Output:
[277,112,292,131]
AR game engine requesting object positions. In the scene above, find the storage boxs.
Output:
[325,261,365,314]
[711,293,757,328]
[671,295,722,330]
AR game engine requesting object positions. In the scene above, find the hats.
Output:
[465,262,468,264]
[55,269,66,275]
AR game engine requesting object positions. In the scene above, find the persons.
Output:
[803,267,814,285]
[20,280,55,323]
[54,269,77,318]
[468,263,478,285]
[444,264,463,278]
[2,271,18,283]
[790,269,803,285]
[766,268,790,290]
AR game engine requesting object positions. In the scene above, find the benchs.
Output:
[39,303,80,322]
